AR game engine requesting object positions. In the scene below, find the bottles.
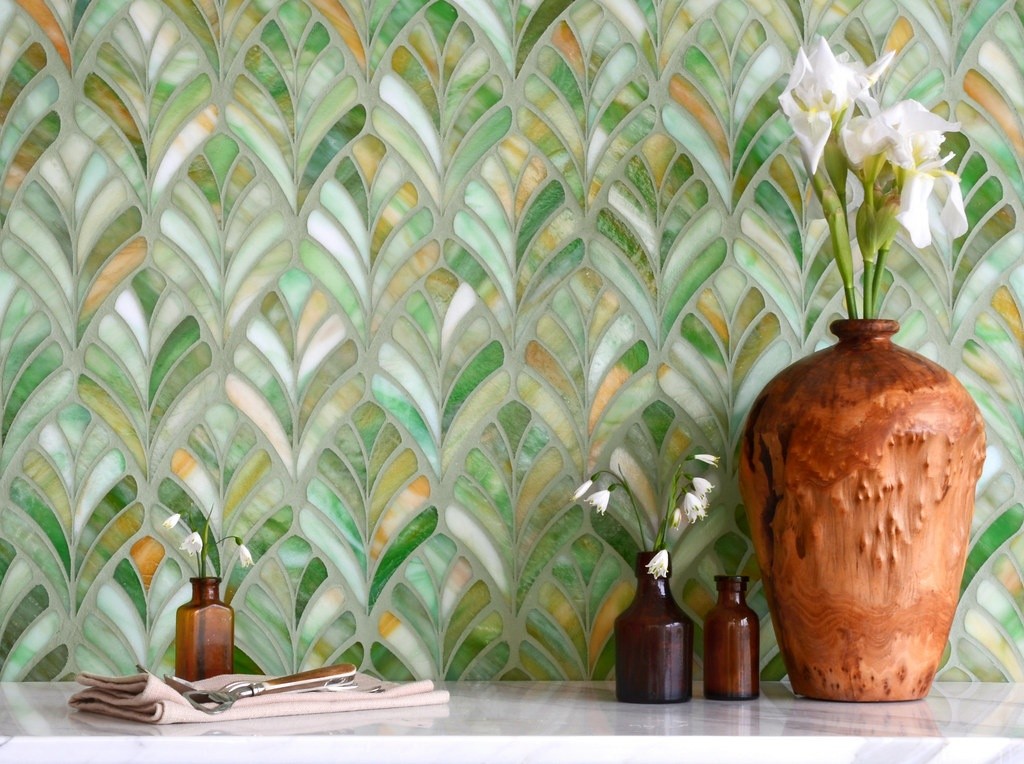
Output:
[704,575,760,701]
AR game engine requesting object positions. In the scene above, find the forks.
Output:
[136,664,359,691]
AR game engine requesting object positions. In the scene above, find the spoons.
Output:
[164,674,381,693]
